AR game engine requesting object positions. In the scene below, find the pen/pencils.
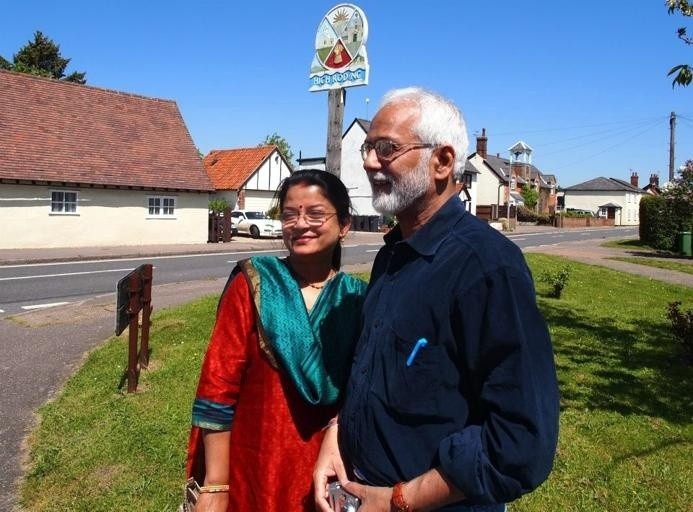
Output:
[406,338,428,366]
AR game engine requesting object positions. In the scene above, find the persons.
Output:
[309,85,563,512]
[185,169,370,512]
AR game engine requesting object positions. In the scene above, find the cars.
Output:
[208,211,238,242]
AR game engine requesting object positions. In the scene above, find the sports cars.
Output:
[214,209,286,239]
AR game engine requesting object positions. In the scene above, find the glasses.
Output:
[282,212,337,227]
[361,141,439,160]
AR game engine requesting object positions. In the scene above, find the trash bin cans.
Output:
[678,232,693,257]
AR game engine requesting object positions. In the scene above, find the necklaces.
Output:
[288,258,338,290]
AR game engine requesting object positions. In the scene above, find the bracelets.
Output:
[199,484,230,494]
[392,480,415,512]
